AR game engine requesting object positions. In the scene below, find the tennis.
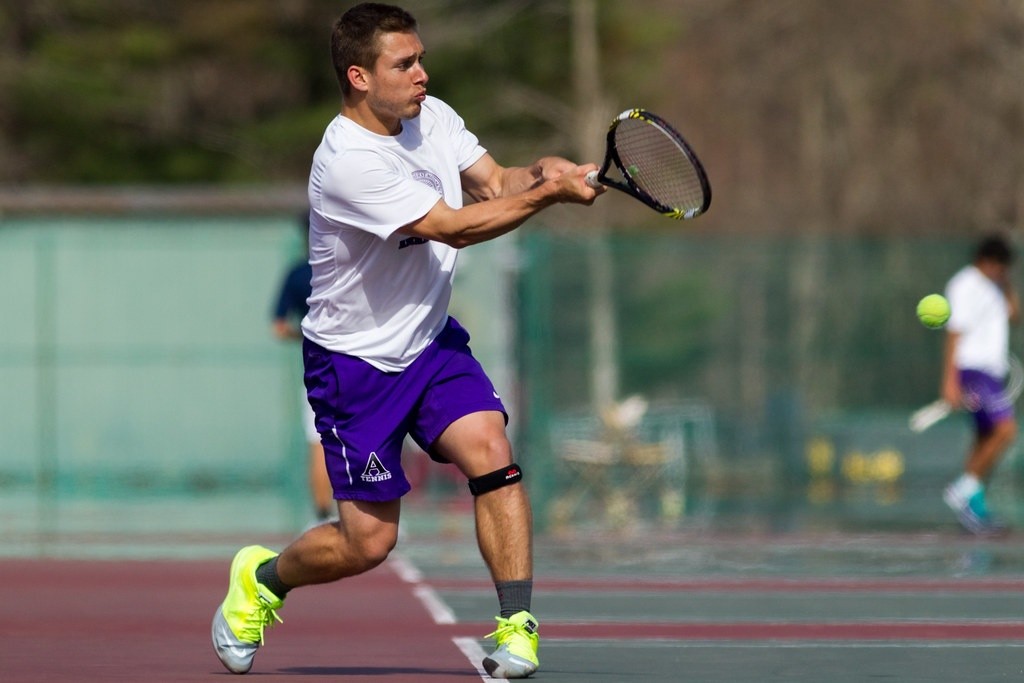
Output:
[916,293,951,328]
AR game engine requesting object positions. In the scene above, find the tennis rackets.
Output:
[586,108,712,220]
[905,351,1024,432]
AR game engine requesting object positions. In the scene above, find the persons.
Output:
[944,233,1022,534]
[273,208,336,530]
[211,1,606,674]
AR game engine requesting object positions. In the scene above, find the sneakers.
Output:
[211,545,286,674]
[483,610,539,678]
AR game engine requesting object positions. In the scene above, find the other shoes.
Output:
[944,482,990,533]
